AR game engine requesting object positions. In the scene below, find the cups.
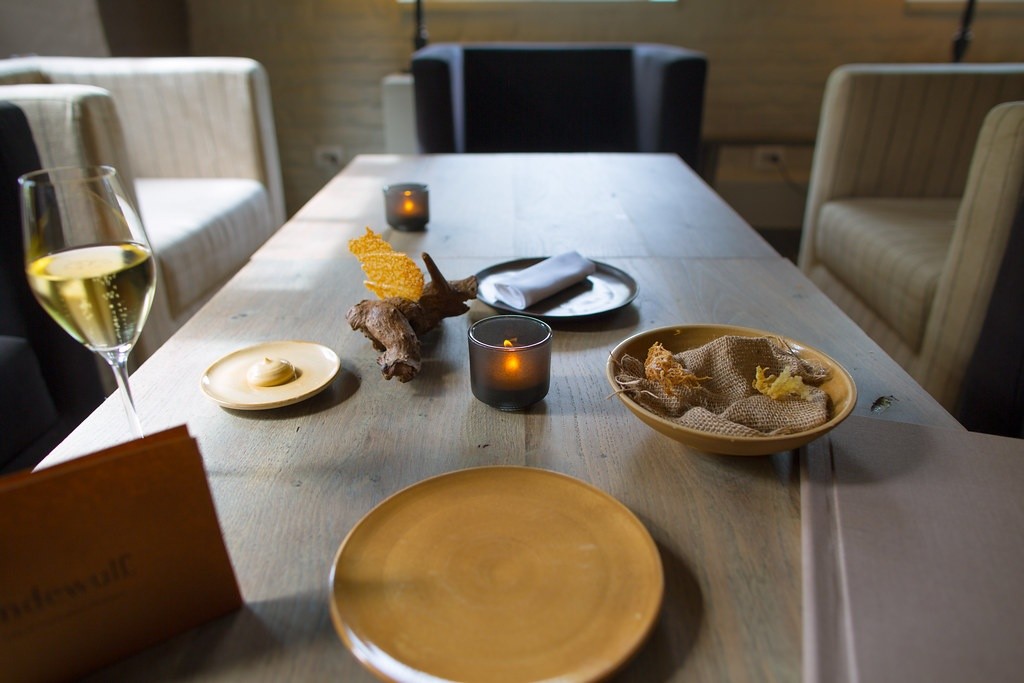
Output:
[466,314,552,412]
[382,183,430,232]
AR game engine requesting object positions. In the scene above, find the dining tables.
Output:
[6,148,1023,681]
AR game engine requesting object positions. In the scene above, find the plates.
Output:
[329,464,666,682]
[200,342,342,410]
[473,255,640,318]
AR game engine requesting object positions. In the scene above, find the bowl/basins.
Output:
[605,324,857,457]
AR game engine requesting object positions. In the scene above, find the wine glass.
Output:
[19,162,158,441]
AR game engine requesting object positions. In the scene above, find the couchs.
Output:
[799,59,1024,438]
[0,96,109,474]
[412,41,710,181]
[0,54,287,379]
